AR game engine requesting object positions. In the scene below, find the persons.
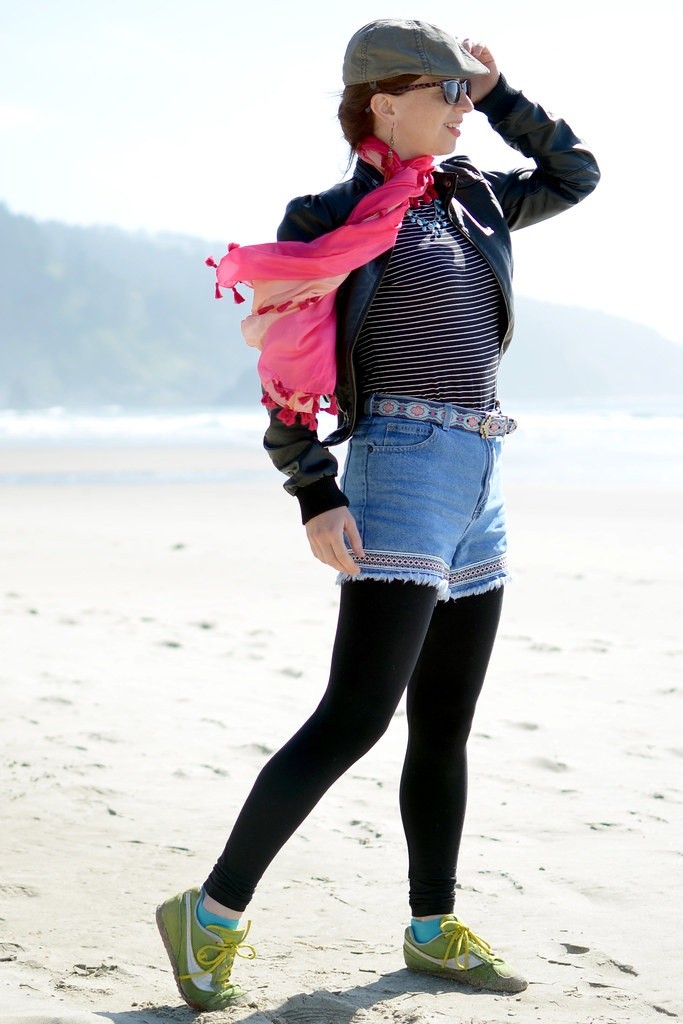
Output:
[156,15,601,1015]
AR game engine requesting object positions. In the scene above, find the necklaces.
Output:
[406,199,445,236]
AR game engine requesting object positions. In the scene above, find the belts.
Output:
[363,399,518,440]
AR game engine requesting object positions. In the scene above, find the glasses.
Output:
[366,79,471,114]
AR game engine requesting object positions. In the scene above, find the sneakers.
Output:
[404,915,528,992]
[156,886,256,1012]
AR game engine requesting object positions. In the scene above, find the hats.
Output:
[342,18,490,85]
